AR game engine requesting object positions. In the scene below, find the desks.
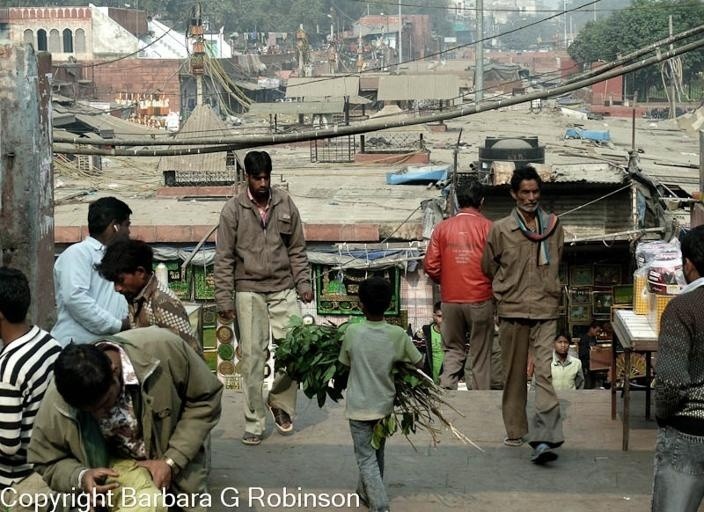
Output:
[609,302,661,453]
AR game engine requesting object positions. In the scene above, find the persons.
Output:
[48,196,133,345]
[332,276,422,512]
[93,239,204,361]
[650,223,704,512]
[0,267,72,512]
[213,151,313,446]
[26,325,224,512]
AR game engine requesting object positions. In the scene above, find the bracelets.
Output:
[163,455,179,475]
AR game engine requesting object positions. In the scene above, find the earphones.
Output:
[113,225,118,231]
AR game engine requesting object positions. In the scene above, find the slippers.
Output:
[503,435,523,447]
[265,403,294,435]
[528,448,559,465]
[240,430,266,446]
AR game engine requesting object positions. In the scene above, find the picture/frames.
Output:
[558,259,624,325]
